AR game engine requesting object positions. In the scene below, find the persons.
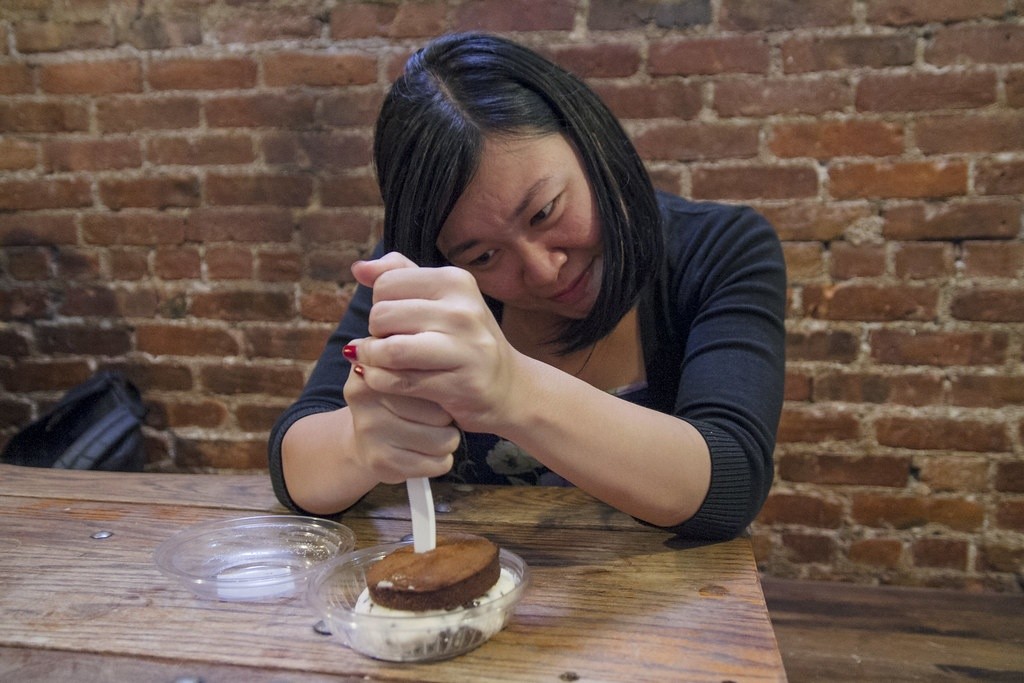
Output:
[268,32,787,540]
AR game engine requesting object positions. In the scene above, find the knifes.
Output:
[404,472,437,556]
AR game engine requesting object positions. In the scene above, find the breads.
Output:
[366,532,504,613]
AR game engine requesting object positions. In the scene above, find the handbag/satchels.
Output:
[1,368,169,473]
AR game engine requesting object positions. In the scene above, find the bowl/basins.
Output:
[305,538,530,664]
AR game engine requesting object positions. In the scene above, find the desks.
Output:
[0,463,790,683]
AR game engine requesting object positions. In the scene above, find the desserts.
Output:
[353,567,518,660]
